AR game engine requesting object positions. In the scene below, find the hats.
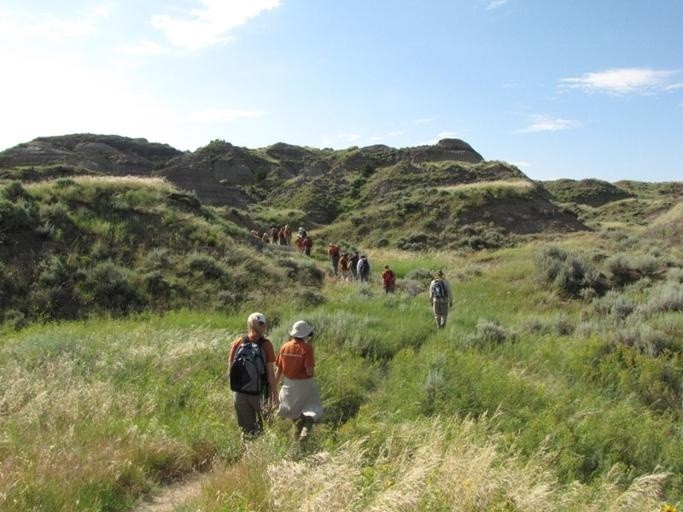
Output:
[247,312,266,324]
[289,320,315,339]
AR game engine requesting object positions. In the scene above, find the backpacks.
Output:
[431,279,447,299]
[229,335,267,396]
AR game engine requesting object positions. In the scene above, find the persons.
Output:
[250,223,372,281]
[428,270,454,330]
[228,313,278,441]
[275,320,324,451]
[381,265,395,294]
